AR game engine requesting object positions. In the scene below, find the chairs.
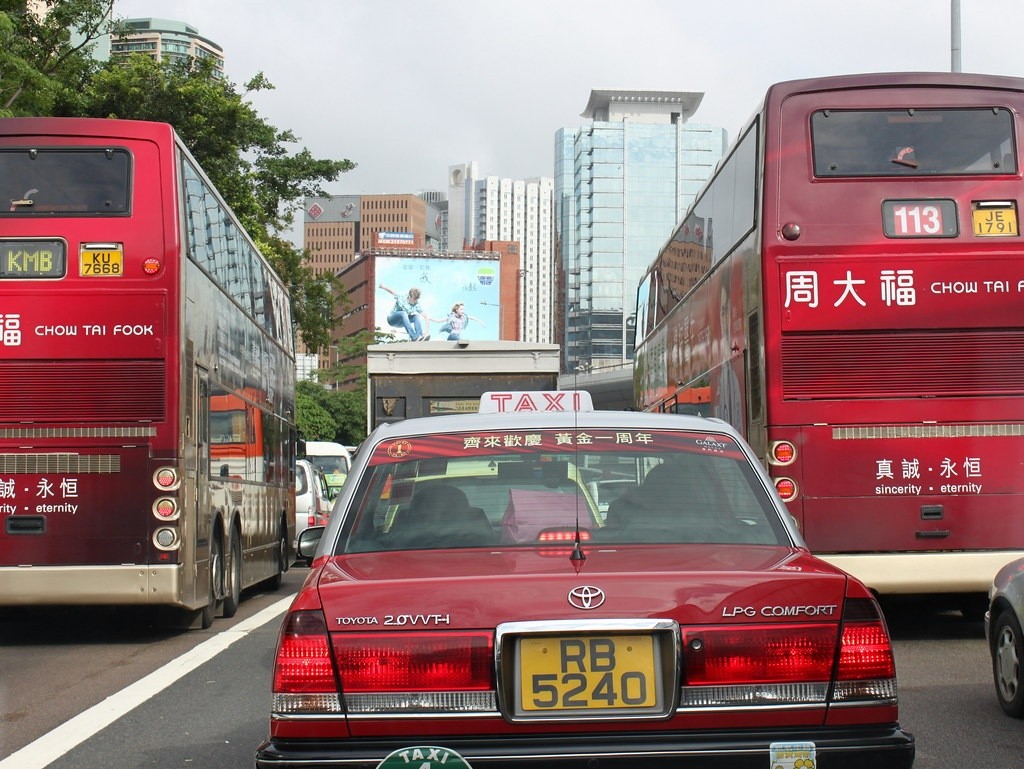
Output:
[386,506,495,550]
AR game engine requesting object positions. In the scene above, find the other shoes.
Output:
[416,336,423,341]
[422,334,431,341]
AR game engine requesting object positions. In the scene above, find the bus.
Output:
[0,114,306,631]
[623,72,1023,631]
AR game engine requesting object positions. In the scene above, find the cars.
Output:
[288,437,642,567]
[984,556,1023,718]
[253,389,915,769]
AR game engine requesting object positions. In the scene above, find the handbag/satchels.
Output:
[498,489,592,543]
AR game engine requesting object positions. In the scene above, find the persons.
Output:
[379,284,485,342]
[712,273,744,439]
